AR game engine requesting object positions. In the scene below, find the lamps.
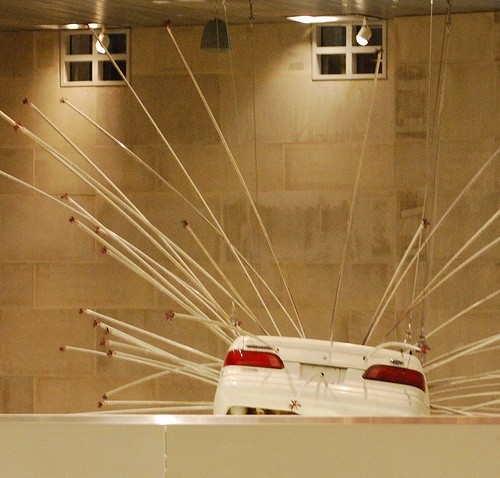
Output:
[356,17,373,46]
[95,24,110,54]
[199,1,231,52]
